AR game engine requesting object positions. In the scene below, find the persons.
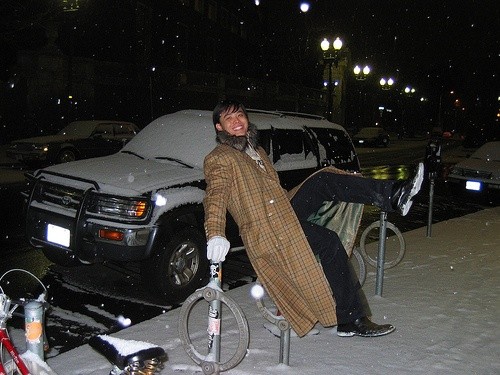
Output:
[203,100,424,336]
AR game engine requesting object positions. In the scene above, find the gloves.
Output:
[207,236,230,263]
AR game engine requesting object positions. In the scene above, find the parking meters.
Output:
[424,139,442,237]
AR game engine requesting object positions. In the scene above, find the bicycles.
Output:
[0,267,170,375]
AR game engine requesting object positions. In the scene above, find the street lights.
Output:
[353,65,370,136]
[438,90,455,124]
[379,77,394,128]
[404,86,416,130]
[320,36,342,121]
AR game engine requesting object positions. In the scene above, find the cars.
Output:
[4,119,140,168]
[353,127,391,148]
[443,138,500,204]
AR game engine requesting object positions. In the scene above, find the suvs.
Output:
[23,108,363,303]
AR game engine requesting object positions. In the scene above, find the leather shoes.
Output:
[336,315,395,337]
[392,162,425,217]
